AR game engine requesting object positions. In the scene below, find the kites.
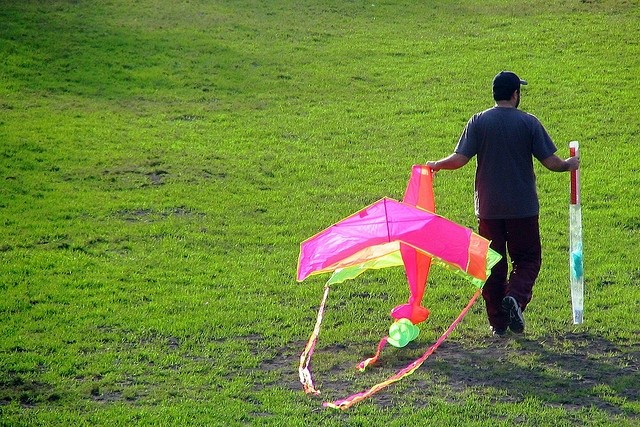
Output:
[295,164,503,411]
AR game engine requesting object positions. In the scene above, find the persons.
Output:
[426,71,581,336]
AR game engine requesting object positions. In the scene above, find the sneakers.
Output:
[490,325,508,338]
[502,296,525,334]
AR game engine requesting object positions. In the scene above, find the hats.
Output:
[493,71,528,88]
